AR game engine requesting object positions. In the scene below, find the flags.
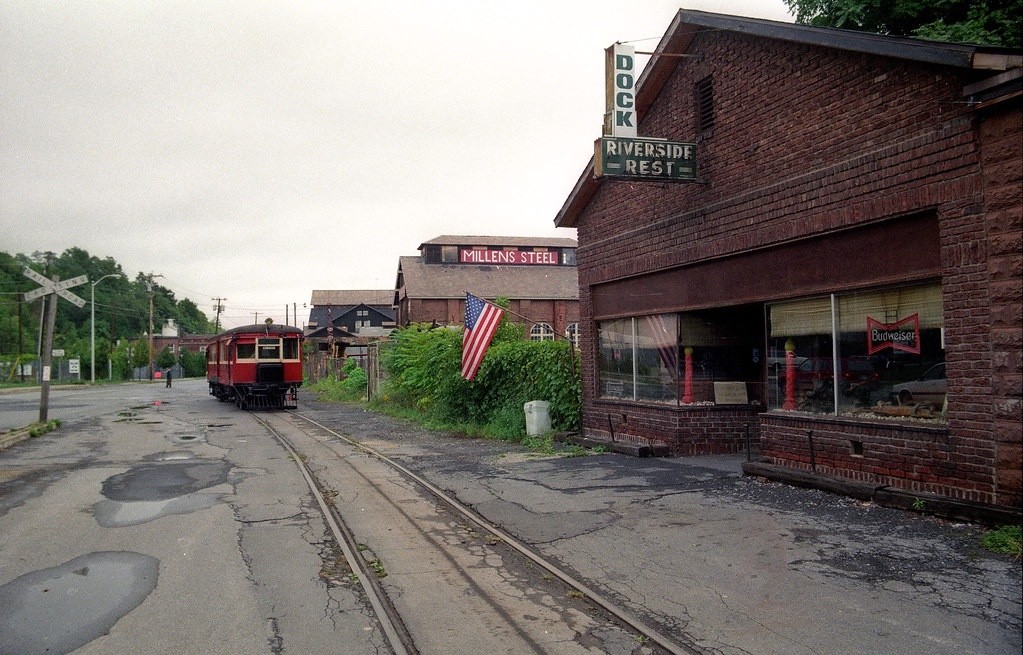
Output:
[645,314,679,379]
[461,293,504,381]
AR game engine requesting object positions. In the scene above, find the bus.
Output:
[204,319,304,411]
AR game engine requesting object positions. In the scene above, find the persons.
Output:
[166,369,172,387]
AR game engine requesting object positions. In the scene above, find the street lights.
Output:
[149,293,174,381]
[91,273,121,384]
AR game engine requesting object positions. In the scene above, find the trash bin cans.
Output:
[523,400,552,437]
